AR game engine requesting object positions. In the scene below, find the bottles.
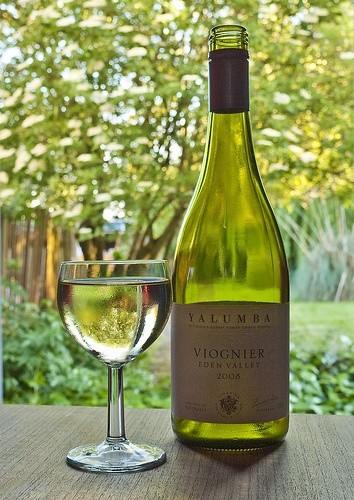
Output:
[171,24,290,454]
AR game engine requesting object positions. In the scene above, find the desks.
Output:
[0,399,354,500]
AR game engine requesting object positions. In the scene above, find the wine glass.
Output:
[56,259,174,474]
